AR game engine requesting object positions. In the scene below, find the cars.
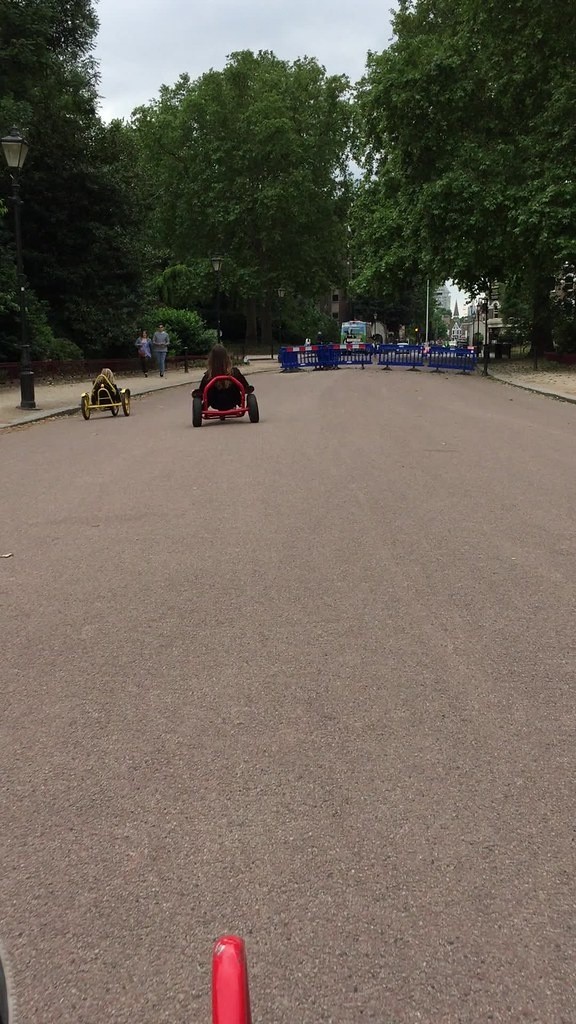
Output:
[395,343,411,354]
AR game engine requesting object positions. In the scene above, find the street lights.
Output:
[414,327,419,345]
[0,124,35,410]
[374,313,377,349]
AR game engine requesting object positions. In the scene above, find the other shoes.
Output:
[160,374,163,377]
[144,373,148,377]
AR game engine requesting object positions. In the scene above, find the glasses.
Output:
[158,327,163,328]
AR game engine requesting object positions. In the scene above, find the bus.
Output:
[342,321,385,349]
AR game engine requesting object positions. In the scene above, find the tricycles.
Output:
[192,375,259,428]
[80,375,131,420]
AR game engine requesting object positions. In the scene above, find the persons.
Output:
[153,324,170,377]
[317,332,324,357]
[191,343,254,411]
[91,368,122,405]
[135,330,152,377]
